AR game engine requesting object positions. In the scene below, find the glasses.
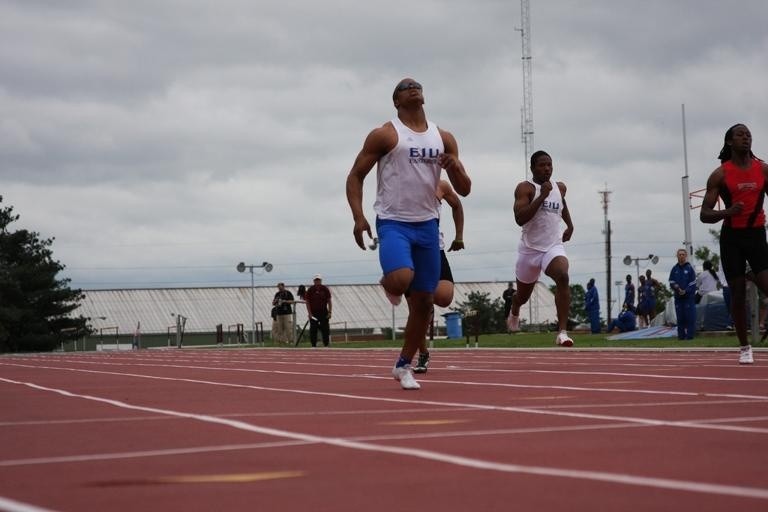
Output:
[396,82,423,91]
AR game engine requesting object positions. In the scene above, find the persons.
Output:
[272,283,294,345]
[306,274,332,348]
[346,78,471,390]
[271,306,286,342]
[503,281,516,335]
[585,249,723,340]
[699,124,768,365]
[414,181,465,372]
[507,151,574,346]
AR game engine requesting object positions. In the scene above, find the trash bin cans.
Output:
[440,312,464,339]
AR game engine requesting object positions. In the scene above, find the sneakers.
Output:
[738,344,754,364]
[413,351,430,374]
[391,361,421,391]
[555,329,574,346]
[507,308,519,332]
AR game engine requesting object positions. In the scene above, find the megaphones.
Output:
[623,255,632,265]
[648,254,659,265]
[235,263,246,273]
[263,262,273,272]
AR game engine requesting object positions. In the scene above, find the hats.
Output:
[314,274,322,281]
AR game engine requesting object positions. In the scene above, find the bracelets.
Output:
[455,240,464,243]
[328,312,331,313]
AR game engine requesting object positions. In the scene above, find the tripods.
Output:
[295,318,323,348]
